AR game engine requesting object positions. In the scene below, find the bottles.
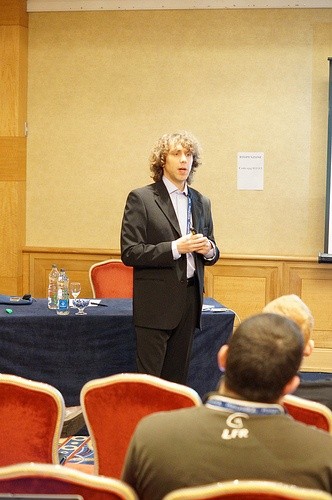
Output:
[56,268,69,315]
[48,264,60,309]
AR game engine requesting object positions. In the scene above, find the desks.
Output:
[0,296,235,436]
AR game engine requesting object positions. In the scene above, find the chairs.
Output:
[0,373,332,500]
[89,259,133,298]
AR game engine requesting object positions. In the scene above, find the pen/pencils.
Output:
[90,302,107,306]
[31,297,37,301]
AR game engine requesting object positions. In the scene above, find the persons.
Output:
[119,311,332,499]
[218,291,332,416]
[118,131,219,396]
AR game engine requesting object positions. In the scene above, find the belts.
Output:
[187,277,194,287]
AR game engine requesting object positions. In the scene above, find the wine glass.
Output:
[70,282,81,308]
[72,298,90,315]
